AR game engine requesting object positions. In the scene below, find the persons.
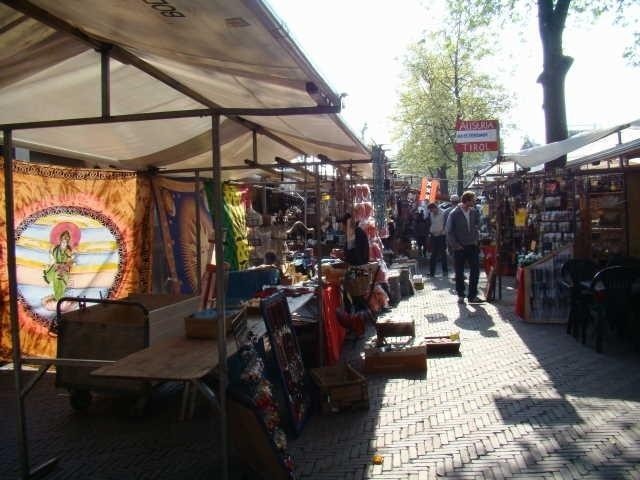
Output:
[442,193,460,219]
[427,203,448,277]
[447,192,487,303]
[330,211,370,265]
[43,230,75,297]
[415,212,427,257]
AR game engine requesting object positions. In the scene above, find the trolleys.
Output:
[54,287,204,413]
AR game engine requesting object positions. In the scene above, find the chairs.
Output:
[560,255,640,352]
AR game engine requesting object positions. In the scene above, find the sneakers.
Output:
[456,295,483,304]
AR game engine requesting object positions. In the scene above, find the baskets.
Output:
[308,361,372,415]
[182,306,239,340]
